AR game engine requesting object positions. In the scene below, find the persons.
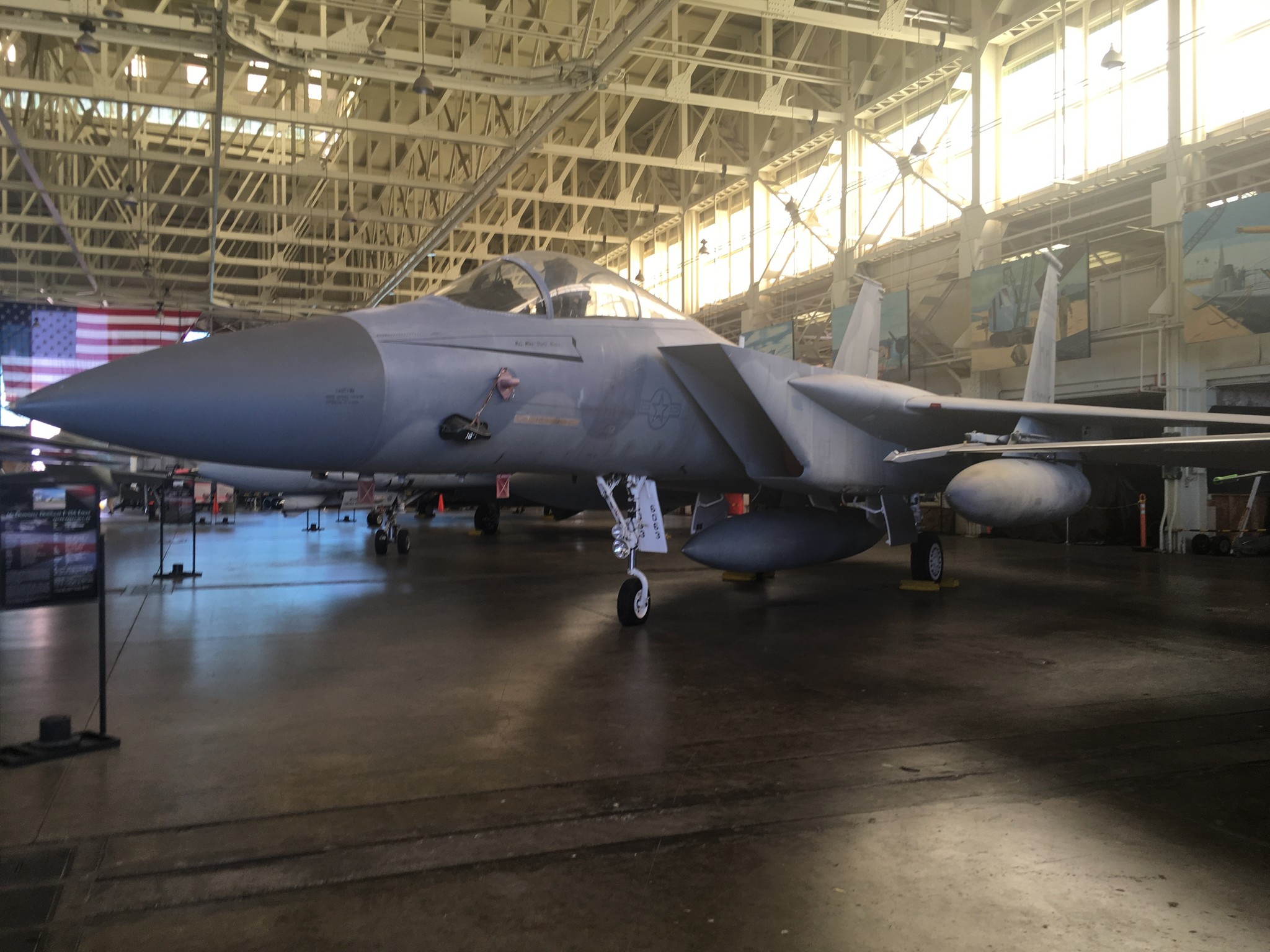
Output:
[107,488,115,514]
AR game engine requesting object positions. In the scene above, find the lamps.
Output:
[71,0,1121,281]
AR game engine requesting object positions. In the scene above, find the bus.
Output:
[0,472,99,599]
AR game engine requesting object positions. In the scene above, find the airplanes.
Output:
[7,248,1270,621]
[173,456,578,557]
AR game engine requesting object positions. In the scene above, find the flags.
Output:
[0,301,202,408]
